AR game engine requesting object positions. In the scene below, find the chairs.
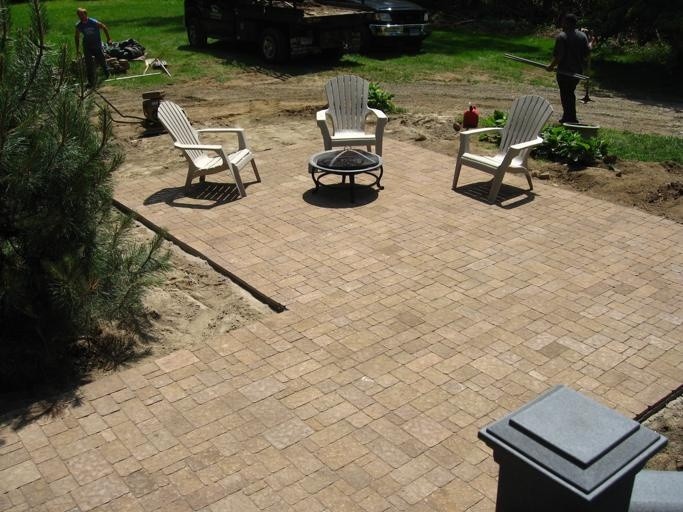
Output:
[316,74,386,157]
[156,100,260,198]
[451,95,553,201]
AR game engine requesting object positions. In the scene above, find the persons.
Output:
[74,7,113,88]
[547,14,593,123]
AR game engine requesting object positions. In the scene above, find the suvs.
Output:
[341,0,431,55]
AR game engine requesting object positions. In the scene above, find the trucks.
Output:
[183,1,374,64]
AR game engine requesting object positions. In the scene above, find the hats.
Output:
[565,13,577,24]
